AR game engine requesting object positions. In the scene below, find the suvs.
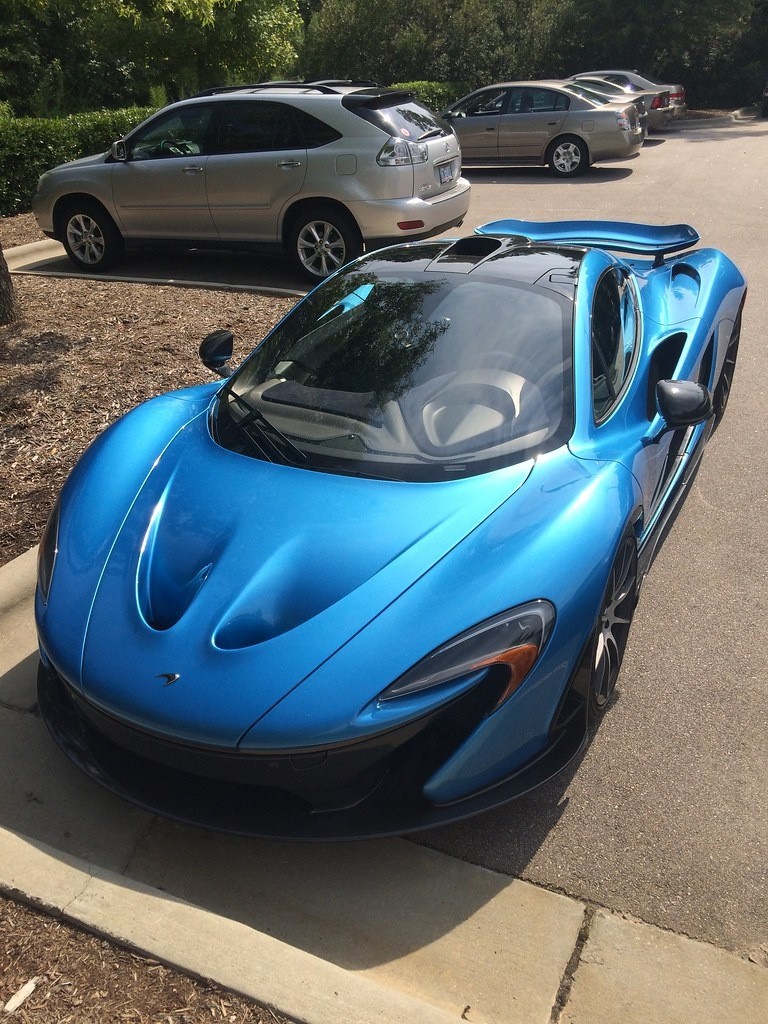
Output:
[29,79,473,286]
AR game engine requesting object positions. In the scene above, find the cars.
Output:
[441,81,646,179]
[537,80,649,140]
[565,68,688,120]
[567,77,676,130]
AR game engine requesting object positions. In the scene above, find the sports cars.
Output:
[31,217,746,847]
